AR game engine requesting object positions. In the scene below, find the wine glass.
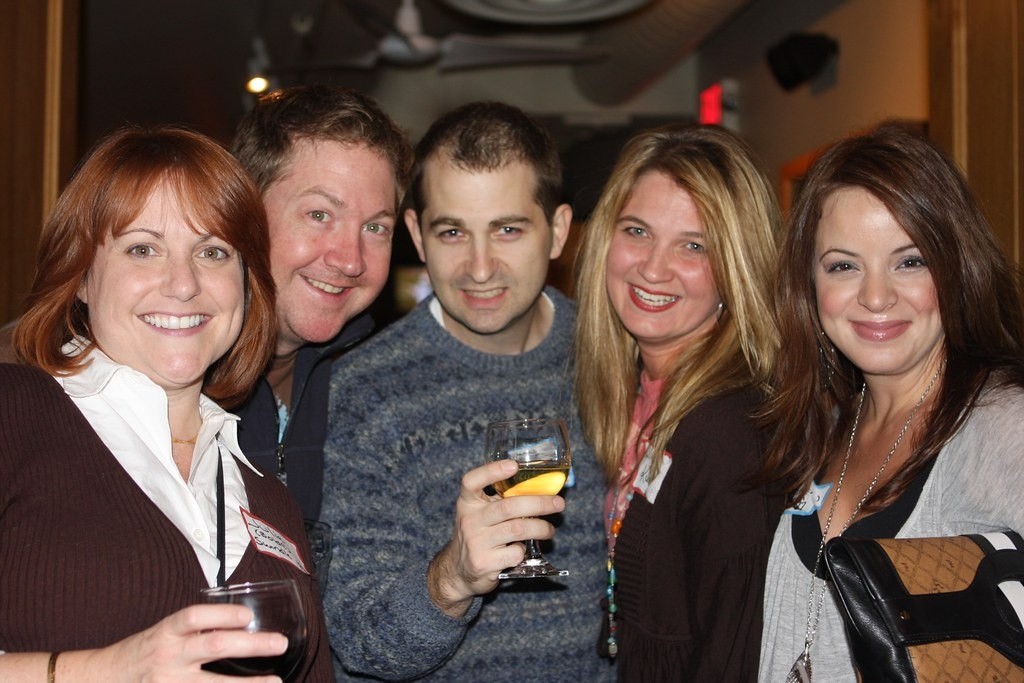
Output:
[484,418,572,579]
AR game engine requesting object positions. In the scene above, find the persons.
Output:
[232,85,405,529]
[575,120,787,683]
[758,131,1024,682]
[318,101,621,683]
[0,128,334,683]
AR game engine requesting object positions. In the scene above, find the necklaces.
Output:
[789,364,948,682]
[172,436,197,446]
[606,462,632,656]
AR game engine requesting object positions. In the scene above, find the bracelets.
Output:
[48,651,60,683]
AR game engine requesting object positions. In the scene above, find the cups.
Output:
[198,579,308,683]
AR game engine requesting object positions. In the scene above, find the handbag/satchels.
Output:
[822,531,1024,683]
